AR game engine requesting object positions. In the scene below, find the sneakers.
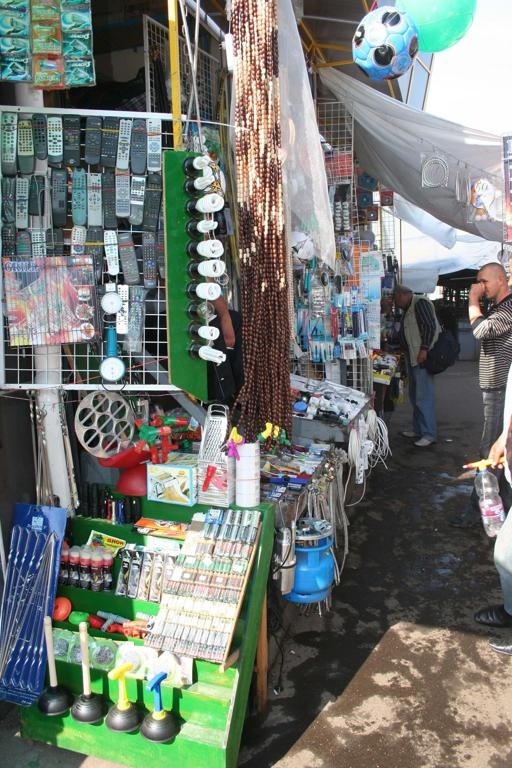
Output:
[414,437,438,447]
[473,604,512,628]
[402,430,421,438]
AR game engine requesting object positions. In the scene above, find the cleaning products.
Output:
[462,456,507,537]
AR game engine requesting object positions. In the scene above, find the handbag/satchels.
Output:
[424,333,461,375]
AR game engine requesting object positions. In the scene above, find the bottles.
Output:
[474,464,506,538]
[59,539,114,593]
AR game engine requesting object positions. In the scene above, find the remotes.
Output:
[0,113,165,353]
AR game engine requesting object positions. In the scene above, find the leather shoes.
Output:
[487,639,512,656]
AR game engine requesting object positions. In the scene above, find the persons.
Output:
[393,285,442,448]
[467,262,512,459]
[205,275,235,397]
[475,258,512,660]
